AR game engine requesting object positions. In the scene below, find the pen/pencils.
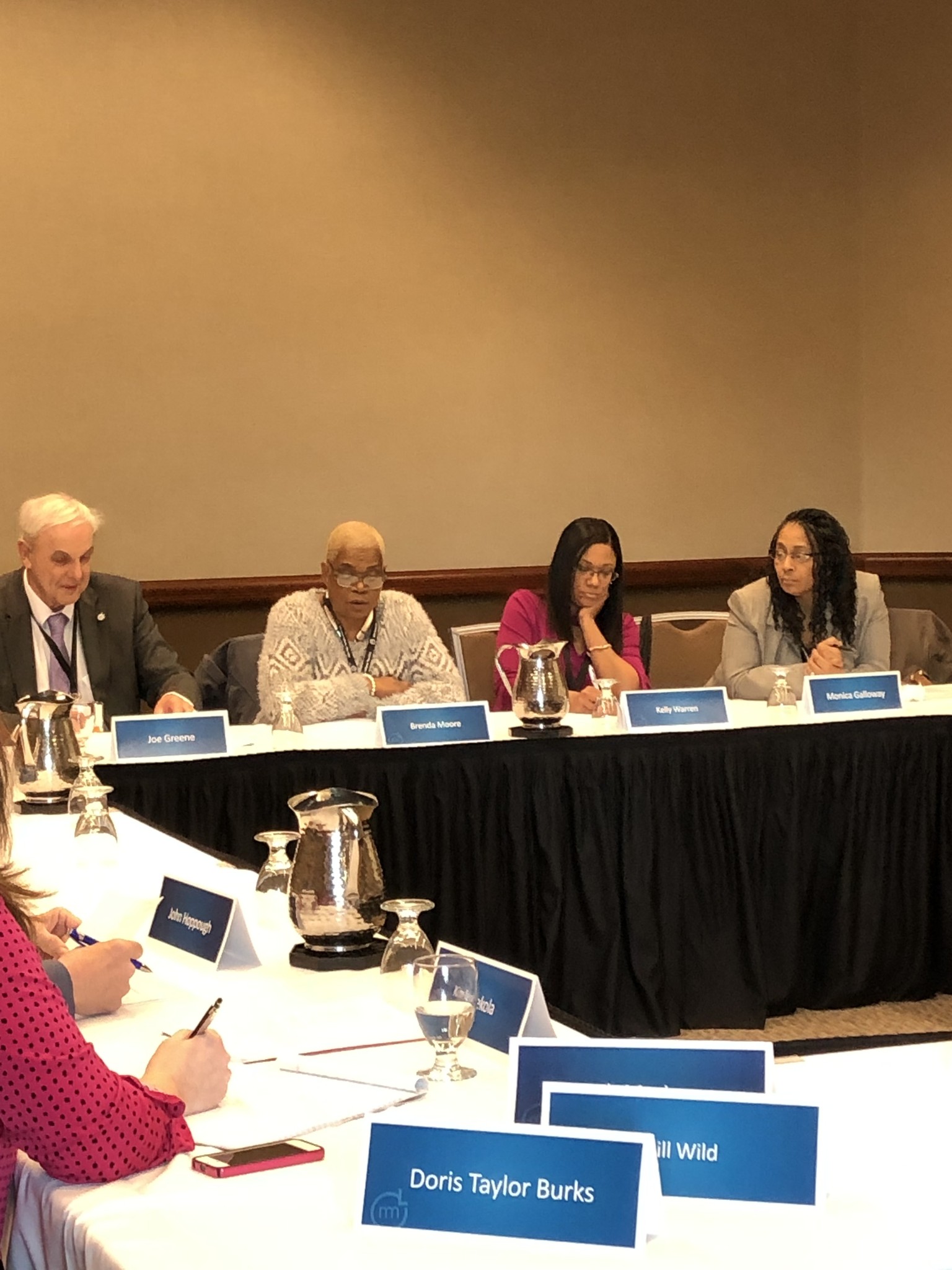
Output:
[588,664,600,690]
[189,997,223,1039]
[63,925,154,974]
[830,642,858,653]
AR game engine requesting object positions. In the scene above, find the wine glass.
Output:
[378,897,437,1010]
[412,953,480,1081]
[270,687,304,748]
[67,754,108,815]
[255,831,303,912]
[74,786,117,847]
[767,667,798,723]
[69,703,94,761]
[592,678,623,733]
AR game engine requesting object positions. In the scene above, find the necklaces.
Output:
[322,591,374,643]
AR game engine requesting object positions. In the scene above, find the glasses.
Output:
[327,558,388,592]
[578,565,619,582]
[768,548,822,564]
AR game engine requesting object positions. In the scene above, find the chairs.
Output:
[634,611,728,690]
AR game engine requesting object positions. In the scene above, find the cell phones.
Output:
[191,1139,324,1178]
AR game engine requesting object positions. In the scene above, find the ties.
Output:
[47,614,73,696]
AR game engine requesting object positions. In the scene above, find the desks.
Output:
[0,684,952,1270]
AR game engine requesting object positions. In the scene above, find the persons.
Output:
[704,508,890,701]
[26,907,143,1020]
[257,520,467,726]
[0,744,233,1253]
[494,517,651,713]
[0,494,199,741]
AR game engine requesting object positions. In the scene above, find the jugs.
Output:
[494,637,569,729]
[287,787,385,954]
[11,689,83,804]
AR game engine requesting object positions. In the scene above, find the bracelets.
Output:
[363,673,376,696]
[589,644,612,651]
